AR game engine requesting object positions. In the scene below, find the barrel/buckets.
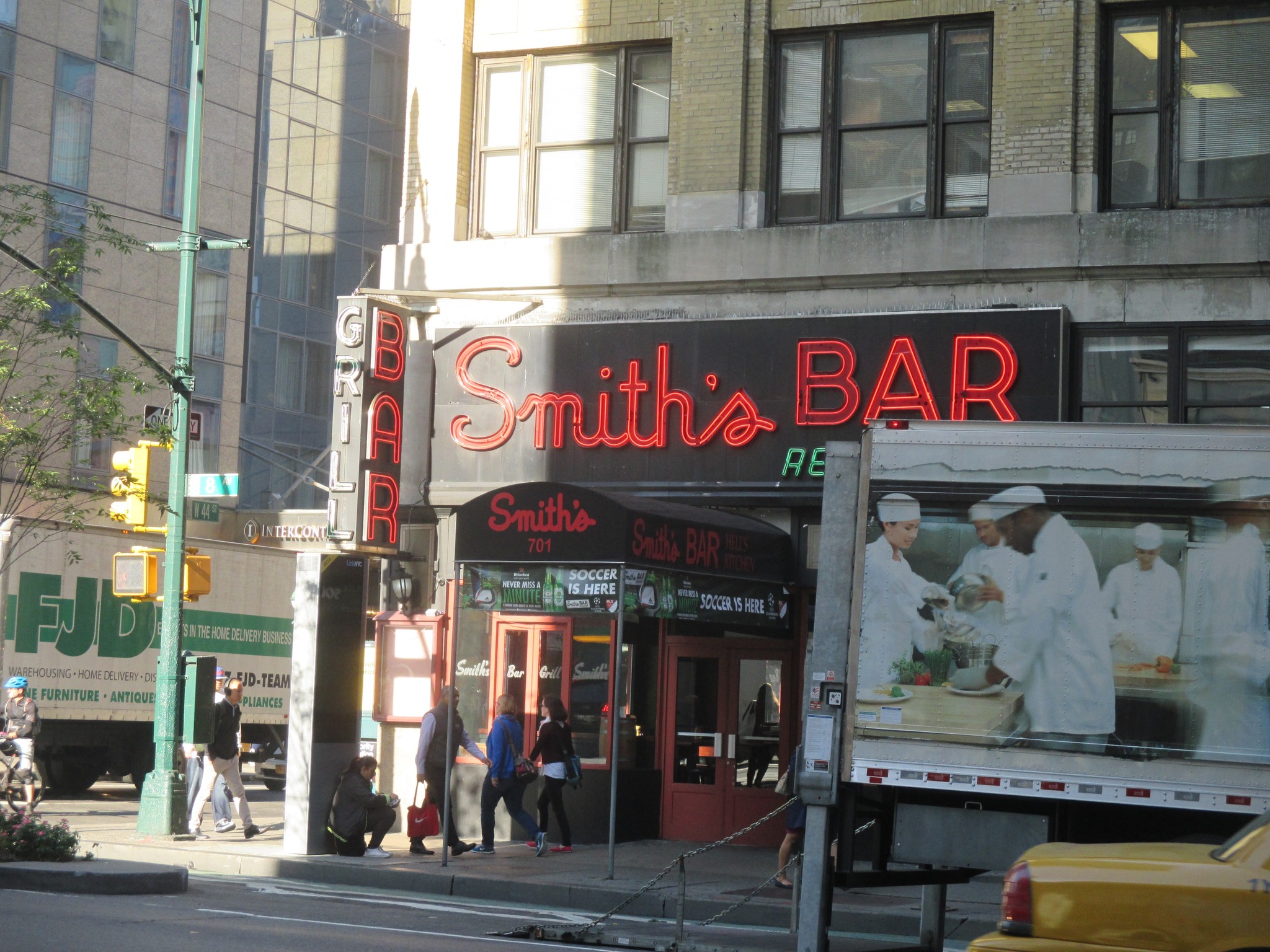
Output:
[599,715,637,771]
[951,634,1000,669]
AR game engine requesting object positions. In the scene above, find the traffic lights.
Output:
[112,552,157,599]
[108,445,151,526]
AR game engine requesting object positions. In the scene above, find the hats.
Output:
[216,666,229,680]
[967,502,992,523]
[989,485,1047,521]
[876,494,921,521]
[1133,523,1164,550]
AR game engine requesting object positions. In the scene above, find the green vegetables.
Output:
[886,656,927,686]
[890,686,905,698]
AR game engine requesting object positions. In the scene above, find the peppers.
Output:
[1155,655,1182,674]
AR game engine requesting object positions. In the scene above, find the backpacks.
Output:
[7,698,41,734]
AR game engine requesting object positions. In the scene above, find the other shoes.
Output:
[452,840,477,856]
[409,843,435,855]
[23,806,33,816]
[775,875,794,889]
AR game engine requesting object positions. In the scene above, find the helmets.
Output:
[4,676,28,689]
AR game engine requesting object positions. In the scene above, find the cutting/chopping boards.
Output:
[854,684,1024,736]
[1113,661,1196,688]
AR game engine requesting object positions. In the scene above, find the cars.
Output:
[960,813,1270,952]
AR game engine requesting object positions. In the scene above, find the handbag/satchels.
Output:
[407,778,440,837]
[566,755,582,786]
[775,771,789,797]
[740,700,757,738]
[515,758,539,783]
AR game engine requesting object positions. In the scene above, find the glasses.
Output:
[495,702,500,706]
[540,704,548,708]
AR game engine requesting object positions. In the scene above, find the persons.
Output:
[773,745,808,890]
[0,676,39,817]
[861,478,1270,764]
[180,666,576,856]
[742,683,779,788]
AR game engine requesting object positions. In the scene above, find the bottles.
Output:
[553,565,565,612]
[543,565,553,612]
[666,575,675,618]
[659,576,668,618]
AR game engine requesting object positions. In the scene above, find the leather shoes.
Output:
[244,824,271,839]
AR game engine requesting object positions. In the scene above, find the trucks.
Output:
[0,515,300,795]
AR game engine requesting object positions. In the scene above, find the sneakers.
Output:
[364,846,393,858]
[191,831,208,838]
[471,845,496,853]
[526,841,537,848]
[549,845,572,853]
[536,832,548,857]
[215,819,236,833]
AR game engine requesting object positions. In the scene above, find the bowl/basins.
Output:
[949,573,989,612]
[942,615,975,638]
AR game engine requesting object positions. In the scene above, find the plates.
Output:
[856,688,913,704]
[946,685,1005,696]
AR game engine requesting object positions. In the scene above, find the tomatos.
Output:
[920,672,930,684]
[915,675,926,686]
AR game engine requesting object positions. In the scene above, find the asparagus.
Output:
[924,648,953,687]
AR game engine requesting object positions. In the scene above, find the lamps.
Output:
[387,562,414,617]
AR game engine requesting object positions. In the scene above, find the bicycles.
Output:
[0,741,47,813]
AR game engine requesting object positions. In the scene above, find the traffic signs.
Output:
[143,403,204,443]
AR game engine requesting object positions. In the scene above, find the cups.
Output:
[921,585,950,610]
